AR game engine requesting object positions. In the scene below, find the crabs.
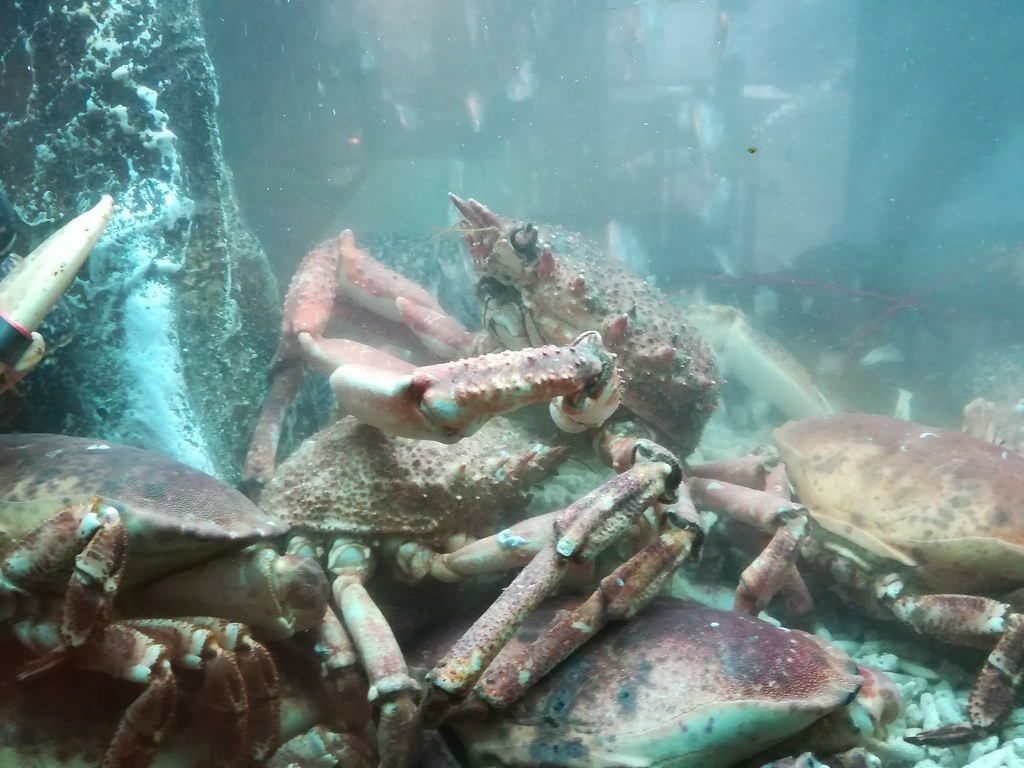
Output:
[688,414,1024,748]
[1,193,331,768]
[239,193,928,768]
